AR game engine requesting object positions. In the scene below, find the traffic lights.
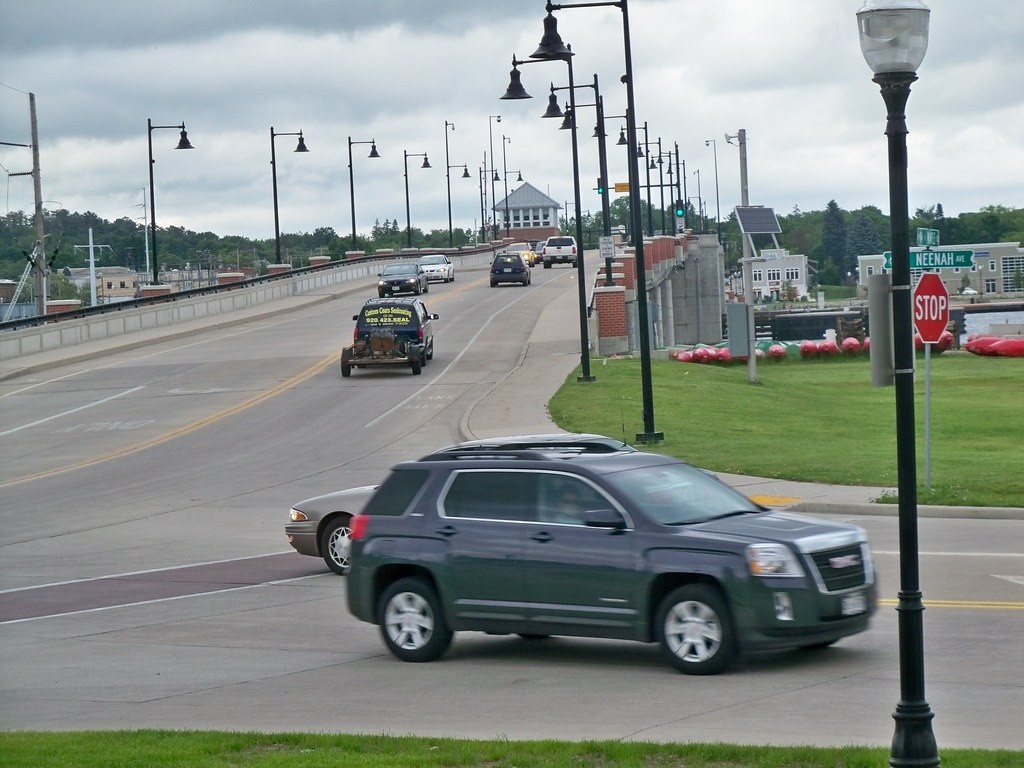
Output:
[597,178,603,194]
[677,200,684,218]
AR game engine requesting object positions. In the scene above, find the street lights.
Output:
[528,0,663,444]
[856,0,939,768]
[348,136,381,251]
[478,167,501,244]
[402,149,432,248]
[502,135,524,237]
[542,75,703,286]
[723,128,786,382]
[498,43,595,383]
[444,120,472,248]
[270,127,310,264]
[148,118,194,285]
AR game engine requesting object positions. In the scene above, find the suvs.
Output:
[490,241,546,287]
[352,298,439,366]
[342,442,878,676]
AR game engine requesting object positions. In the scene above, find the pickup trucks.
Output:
[543,236,578,268]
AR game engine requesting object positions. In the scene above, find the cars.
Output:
[419,255,454,283]
[377,263,428,298]
[286,431,719,592]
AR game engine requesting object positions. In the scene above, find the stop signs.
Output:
[914,273,949,344]
